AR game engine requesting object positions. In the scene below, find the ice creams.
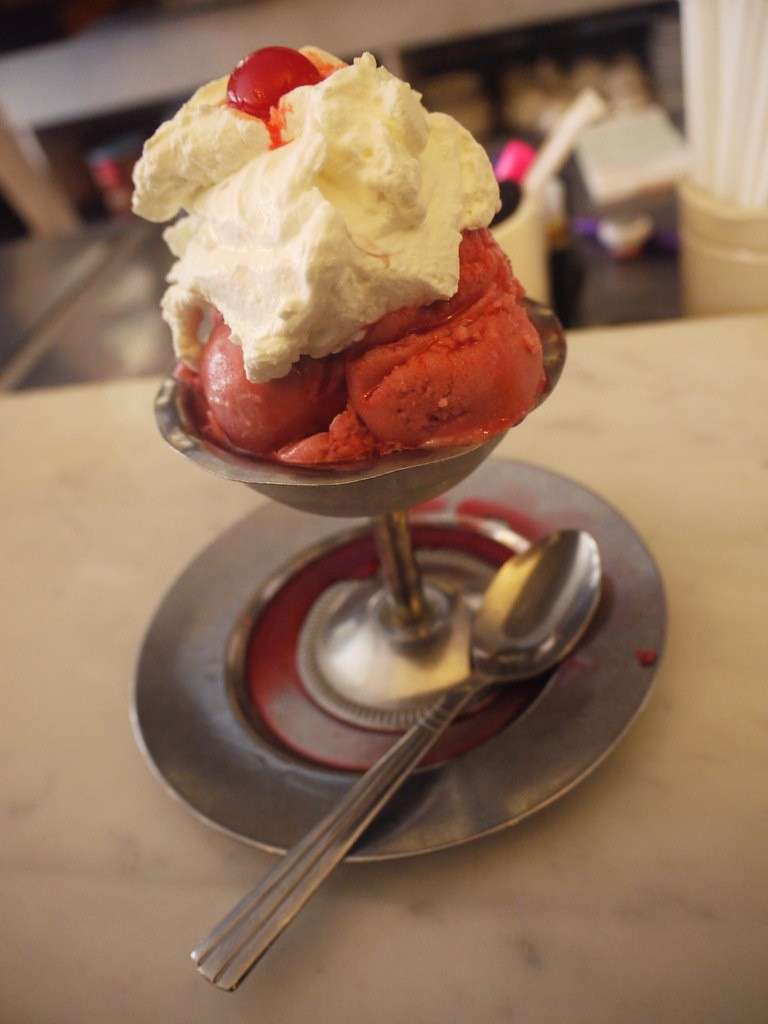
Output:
[130,44,548,468]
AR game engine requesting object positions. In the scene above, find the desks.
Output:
[0,317,768,1024]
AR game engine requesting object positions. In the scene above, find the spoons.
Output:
[189,527,602,990]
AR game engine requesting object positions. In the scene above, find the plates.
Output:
[128,457,668,863]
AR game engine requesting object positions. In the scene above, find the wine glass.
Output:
[154,297,568,728]
